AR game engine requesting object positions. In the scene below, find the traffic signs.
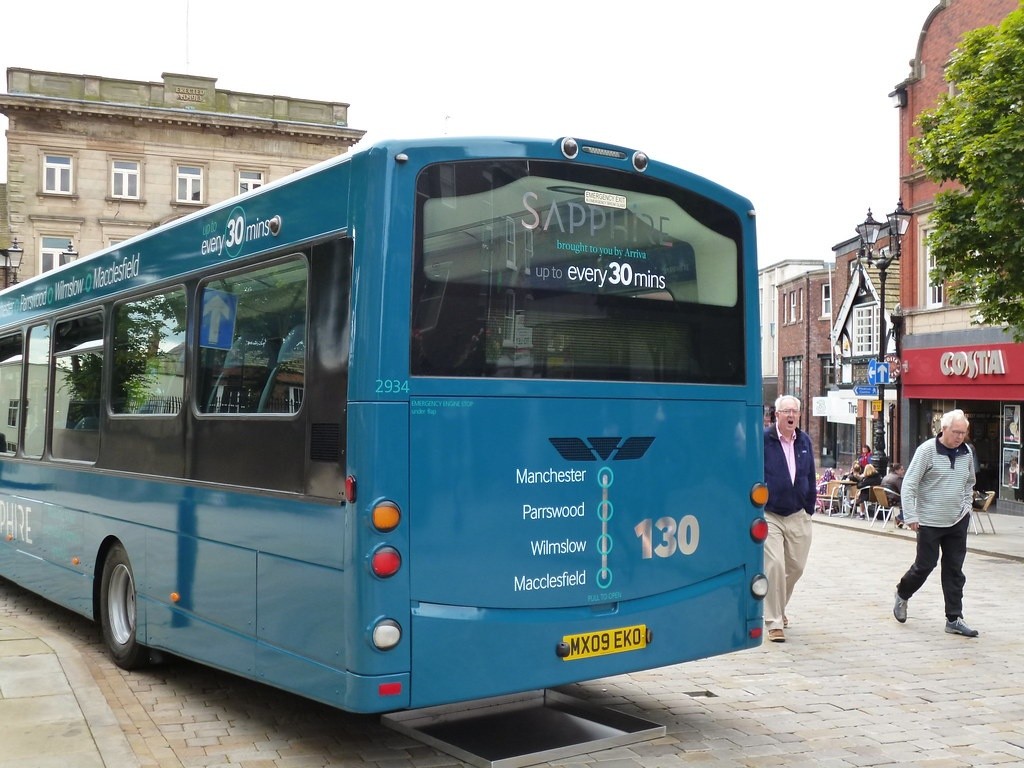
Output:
[853,384,878,397]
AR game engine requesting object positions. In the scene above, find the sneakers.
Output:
[893,590,909,623]
[944,617,978,637]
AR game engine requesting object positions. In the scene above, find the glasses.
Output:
[952,430,969,437]
[778,409,799,415]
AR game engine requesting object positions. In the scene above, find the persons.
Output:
[881,463,904,528]
[1009,457,1019,486]
[857,445,873,471]
[764,395,817,642]
[893,408,978,636]
[964,434,980,489]
[871,442,888,476]
[1006,434,1018,442]
[838,461,881,518]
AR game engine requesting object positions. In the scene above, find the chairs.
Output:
[815,479,997,535]
[74,322,306,431]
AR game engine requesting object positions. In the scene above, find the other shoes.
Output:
[782,615,789,628]
[897,522,904,529]
[856,513,865,520]
[767,629,786,642]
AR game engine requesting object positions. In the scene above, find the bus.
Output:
[0,132,770,718]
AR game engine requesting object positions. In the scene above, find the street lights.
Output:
[854,193,916,475]
